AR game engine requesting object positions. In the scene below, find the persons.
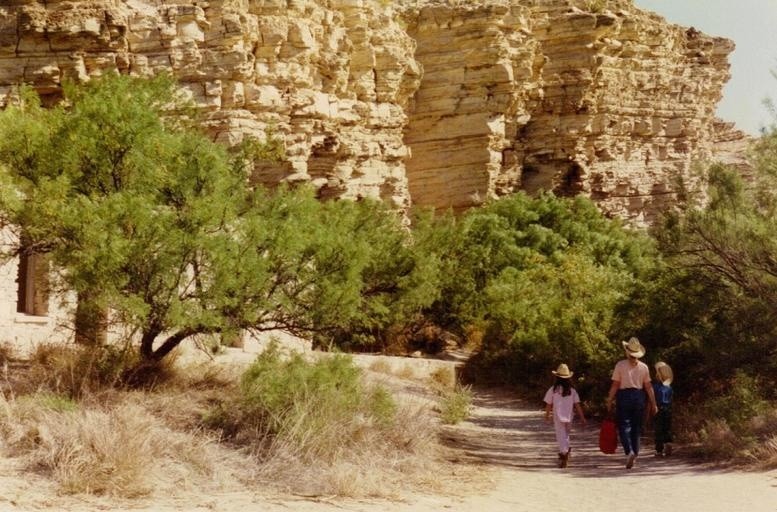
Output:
[607,337,658,469]
[653,362,674,457]
[542,363,588,468]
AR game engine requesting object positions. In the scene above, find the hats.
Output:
[654,359,674,388]
[551,362,574,378]
[620,337,646,358]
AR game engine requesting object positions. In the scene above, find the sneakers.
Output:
[626,450,640,469]
[654,442,673,458]
[558,446,571,469]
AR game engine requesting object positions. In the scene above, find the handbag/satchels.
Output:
[599,418,618,455]
